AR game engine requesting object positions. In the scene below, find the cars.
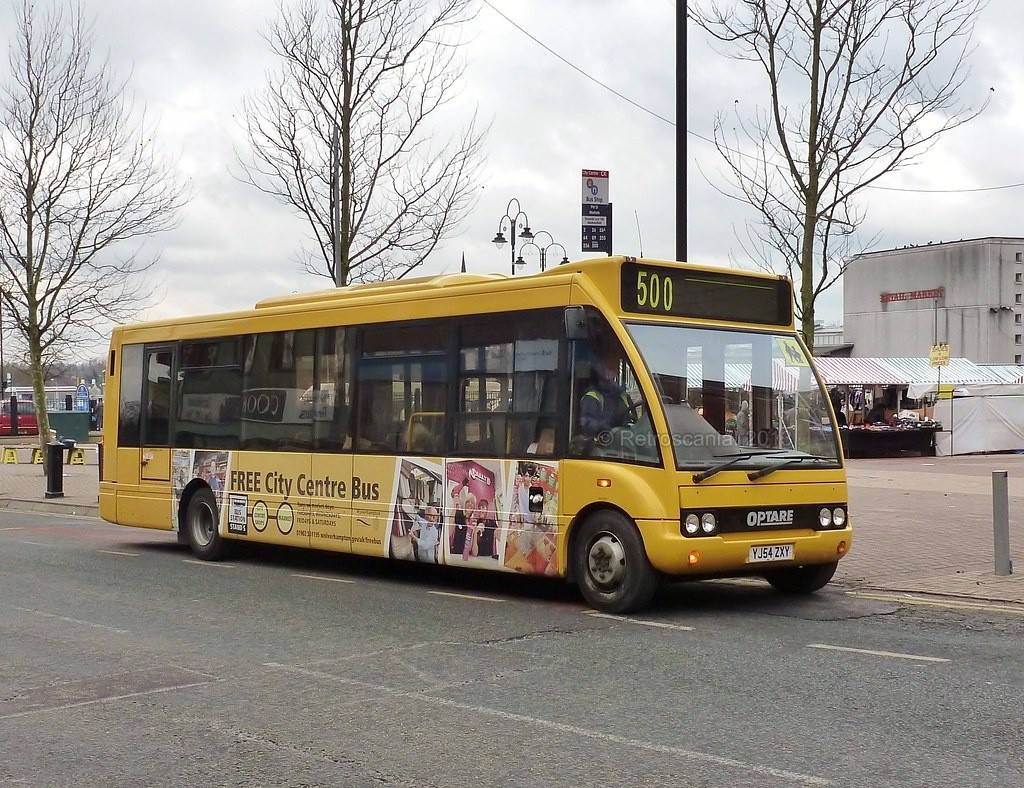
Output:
[0,399,39,436]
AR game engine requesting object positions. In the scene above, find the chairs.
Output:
[533,359,593,457]
[397,422,437,455]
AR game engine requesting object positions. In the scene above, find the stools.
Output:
[30,449,43,465]
[1,448,18,465]
[67,449,86,465]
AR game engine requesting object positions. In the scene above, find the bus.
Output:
[99,255,855,617]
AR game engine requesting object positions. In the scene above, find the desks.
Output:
[810,425,944,459]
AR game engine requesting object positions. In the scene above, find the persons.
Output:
[175,470,186,532]
[517,467,545,531]
[450,493,497,557]
[207,459,222,495]
[736,401,748,446]
[579,360,638,436]
[840,399,854,426]
[405,507,439,564]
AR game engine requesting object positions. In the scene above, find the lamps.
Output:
[990,305,1014,314]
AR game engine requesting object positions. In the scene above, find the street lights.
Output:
[492,198,535,277]
[511,230,569,274]
